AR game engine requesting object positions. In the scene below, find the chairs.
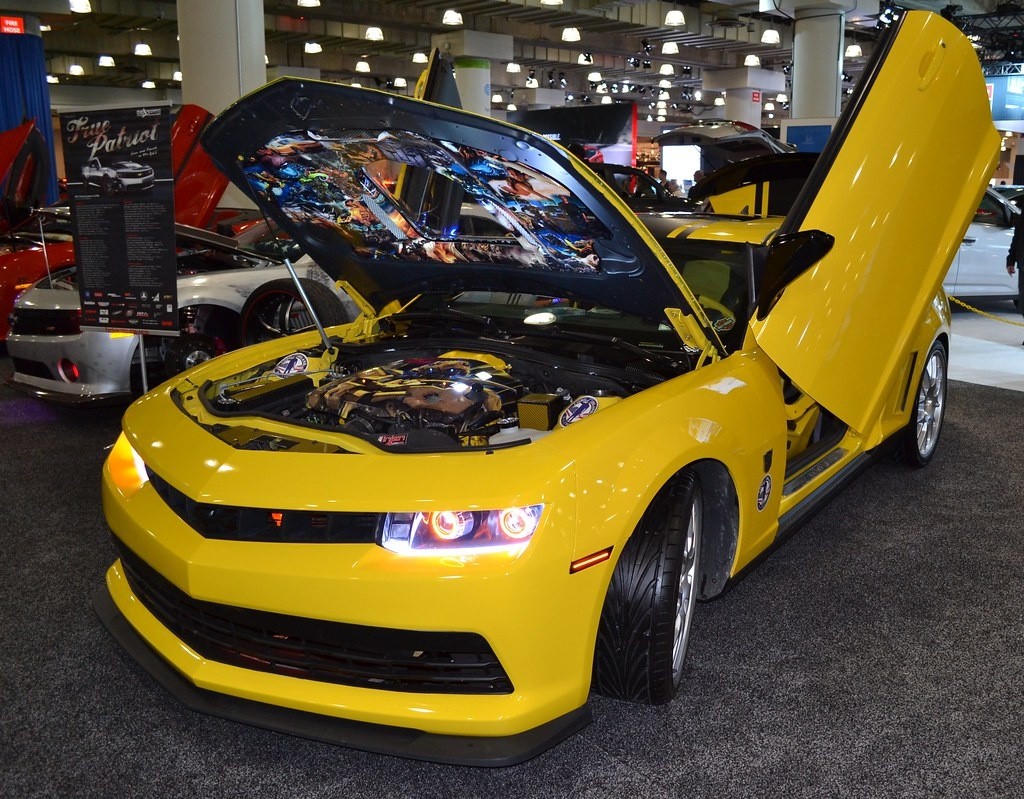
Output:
[684,259,741,315]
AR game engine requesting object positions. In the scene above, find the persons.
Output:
[1006,206,1024,346]
[658,168,704,202]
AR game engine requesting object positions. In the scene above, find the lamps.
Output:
[46,0,862,123]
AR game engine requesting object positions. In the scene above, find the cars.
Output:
[940,188,1024,301]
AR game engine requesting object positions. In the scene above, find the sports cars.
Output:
[5,122,796,407]
[1,101,291,347]
[101,10,1003,771]
